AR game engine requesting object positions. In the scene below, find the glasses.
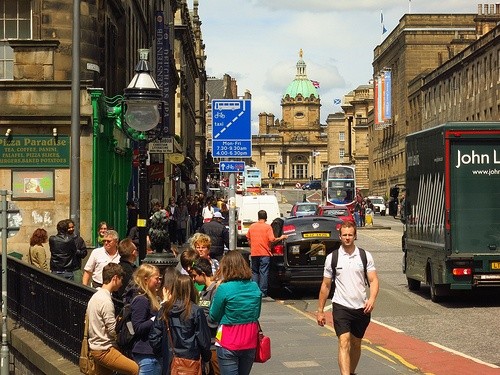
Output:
[192,262,203,272]
[102,239,114,243]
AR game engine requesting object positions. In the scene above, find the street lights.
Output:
[122,49,164,266]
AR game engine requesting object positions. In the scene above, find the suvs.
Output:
[364,196,386,216]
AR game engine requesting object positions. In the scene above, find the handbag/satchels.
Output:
[78,339,102,375]
[254,334,271,364]
[171,358,202,375]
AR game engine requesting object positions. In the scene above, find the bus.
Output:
[322,165,357,214]
[243,167,261,195]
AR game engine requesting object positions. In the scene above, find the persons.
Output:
[190,257,221,375]
[22,227,48,272]
[126,172,229,302]
[82,229,121,290]
[245,210,288,298]
[351,198,376,227]
[397,188,406,218]
[122,262,162,375]
[97,221,113,248]
[206,250,262,375]
[65,219,88,270]
[85,262,139,375]
[112,238,138,302]
[316,221,379,375]
[48,220,81,281]
[156,274,212,375]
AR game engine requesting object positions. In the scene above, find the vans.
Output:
[302,180,321,190]
[236,195,283,247]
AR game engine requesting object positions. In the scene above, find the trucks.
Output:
[389,122,500,303]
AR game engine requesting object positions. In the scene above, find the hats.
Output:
[213,212,226,220]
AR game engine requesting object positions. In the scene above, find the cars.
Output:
[314,206,357,240]
[287,202,320,216]
[268,215,345,298]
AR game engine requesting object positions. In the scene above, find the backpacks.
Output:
[115,294,151,350]
